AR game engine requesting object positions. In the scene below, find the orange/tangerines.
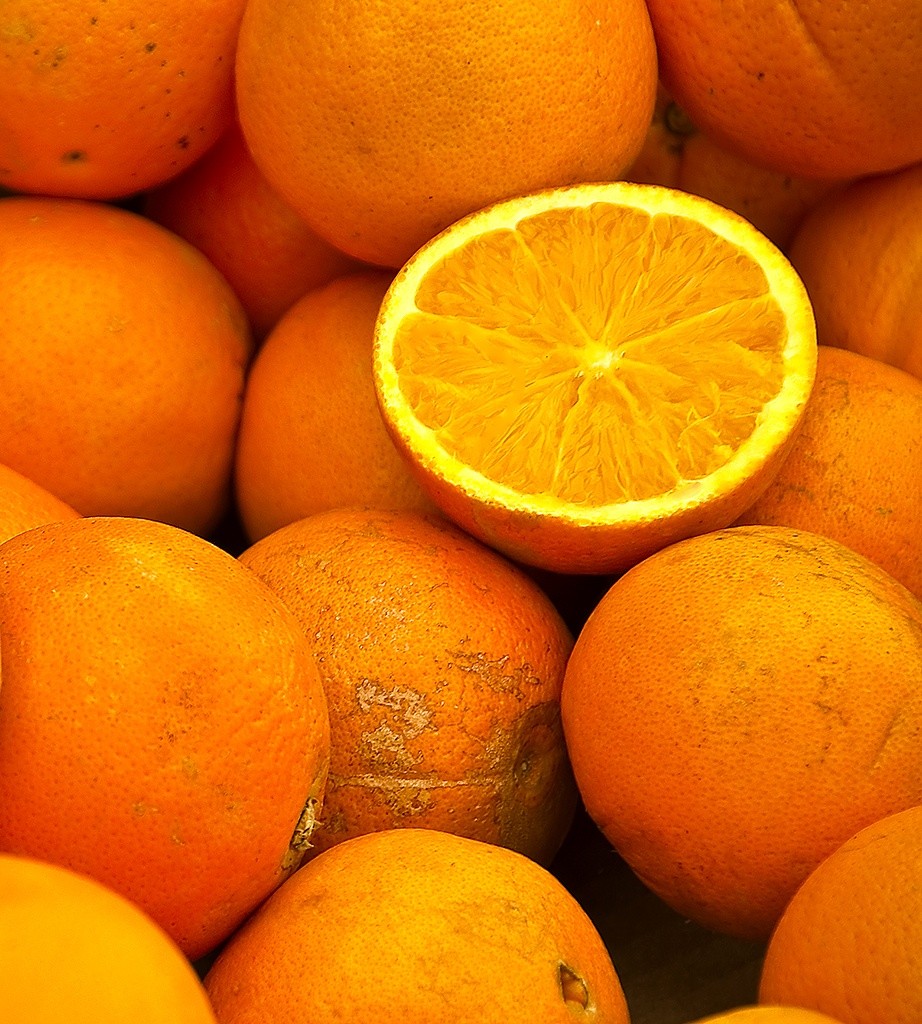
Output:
[0,1,922,1024]
